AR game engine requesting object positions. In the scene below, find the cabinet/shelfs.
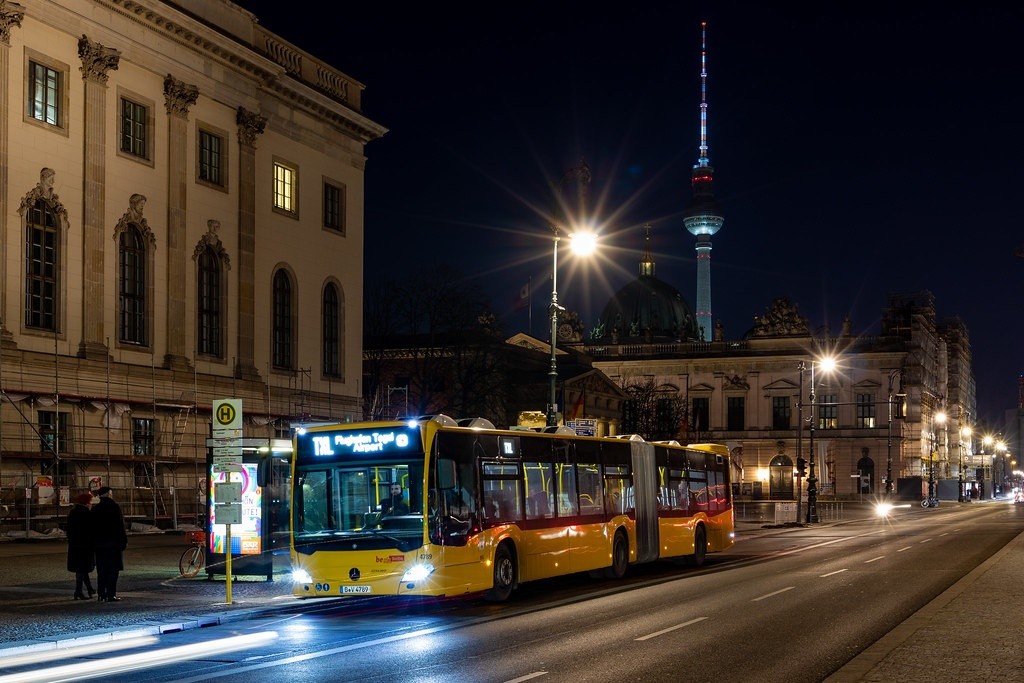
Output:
[0,486,204,539]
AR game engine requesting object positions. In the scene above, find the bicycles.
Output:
[178,512,208,579]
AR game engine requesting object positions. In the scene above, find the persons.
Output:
[422,489,466,517]
[66,494,93,599]
[379,482,409,524]
[486,500,515,521]
[90,486,128,602]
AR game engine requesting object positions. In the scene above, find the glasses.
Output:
[391,488,398,490]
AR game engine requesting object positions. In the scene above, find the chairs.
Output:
[579,494,596,514]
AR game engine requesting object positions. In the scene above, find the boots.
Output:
[88,586,96,597]
[73,588,85,600]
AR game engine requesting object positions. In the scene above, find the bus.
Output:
[256,414,736,607]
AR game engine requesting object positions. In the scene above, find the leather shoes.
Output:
[98,593,122,602]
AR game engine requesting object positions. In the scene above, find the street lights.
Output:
[546,220,599,427]
[805,353,839,524]
[958,425,974,502]
[980,434,995,500]
[993,440,1008,498]
[886,367,907,504]
[929,411,949,499]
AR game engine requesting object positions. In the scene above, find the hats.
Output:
[98,487,113,496]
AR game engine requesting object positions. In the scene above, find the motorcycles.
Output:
[920,494,940,509]
